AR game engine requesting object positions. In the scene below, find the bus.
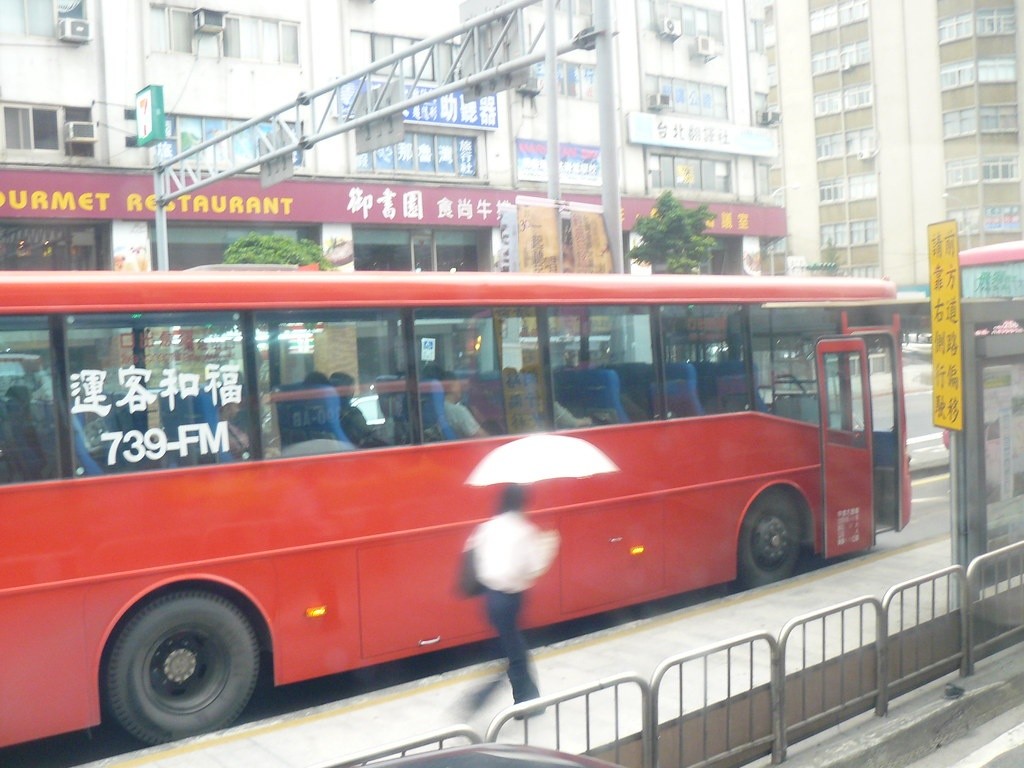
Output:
[0,263,912,750]
[943,241,1024,451]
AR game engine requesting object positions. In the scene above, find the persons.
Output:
[521,364,593,428]
[219,403,250,457]
[434,371,489,437]
[305,373,387,449]
[452,487,560,721]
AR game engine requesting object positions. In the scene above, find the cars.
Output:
[809,345,950,473]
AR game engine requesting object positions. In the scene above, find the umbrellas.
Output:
[464,434,619,487]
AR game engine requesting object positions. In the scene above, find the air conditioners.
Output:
[648,93,671,107]
[762,111,780,124]
[64,121,99,143]
[194,10,226,33]
[857,149,870,161]
[59,17,92,43]
[694,35,713,57]
[515,76,542,96]
[659,16,682,37]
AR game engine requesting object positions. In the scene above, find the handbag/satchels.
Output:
[460,549,485,596]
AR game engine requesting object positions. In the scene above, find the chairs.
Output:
[648,362,704,418]
[579,368,629,426]
[113,391,161,472]
[715,360,767,414]
[8,400,46,480]
[29,400,103,478]
[277,383,354,455]
[619,362,653,414]
[158,388,232,468]
[100,406,131,472]
[471,372,543,437]
[554,365,584,420]
[376,375,406,421]
[404,378,458,443]
